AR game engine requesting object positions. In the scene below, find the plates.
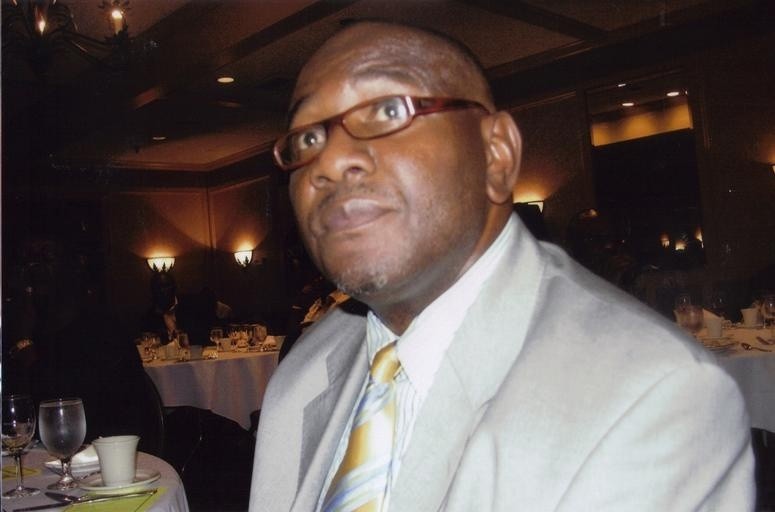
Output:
[736,323,763,329]
[77,468,163,495]
[700,333,733,340]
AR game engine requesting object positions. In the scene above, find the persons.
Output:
[140,272,213,341]
[205,287,237,324]
[289,259,350,329]
[248,14,757,512]
[2,264,159,451]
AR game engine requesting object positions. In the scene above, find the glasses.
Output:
[270,95,490,170]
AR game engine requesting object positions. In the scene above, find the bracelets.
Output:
[9,337,36,359]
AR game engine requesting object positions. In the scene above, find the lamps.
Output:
[234,250,254,269]
[2,0,138,95]
[144,256,175,274]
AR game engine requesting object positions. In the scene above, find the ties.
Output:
[320,341,401,512]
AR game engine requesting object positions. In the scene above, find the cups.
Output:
[704,316,724,339]
[740,308,759,325]
[91,436,141,487]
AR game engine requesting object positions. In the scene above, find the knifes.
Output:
[13,489,157,512]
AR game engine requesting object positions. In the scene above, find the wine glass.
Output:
[138,323,267,361]
[0,394,88,498]
